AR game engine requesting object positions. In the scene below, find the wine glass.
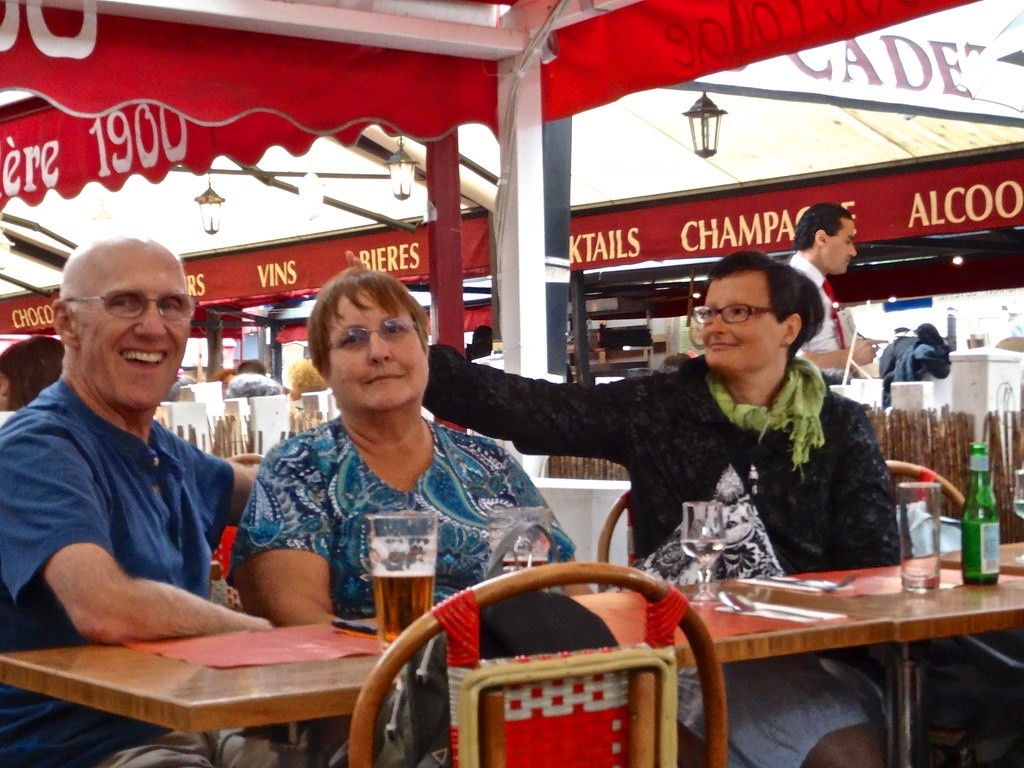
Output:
[678,500,728,603]
[1013,469,1024,560]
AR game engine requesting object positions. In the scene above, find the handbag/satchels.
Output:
[325,521,624,768]
[879,322,951,407]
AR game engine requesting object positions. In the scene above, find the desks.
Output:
[939,542,1024,576]
[715,566,1024,768]
[0,586,894,768]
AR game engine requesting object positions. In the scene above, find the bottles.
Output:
[961,442,1001,585]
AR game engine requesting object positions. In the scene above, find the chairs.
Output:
[885,459,965,552]
[347,560,727,768]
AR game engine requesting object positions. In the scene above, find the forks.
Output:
[758,572,862,591]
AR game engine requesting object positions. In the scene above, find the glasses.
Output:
[327,317,418,352]
[691,302,772,324]
[64,288,199,322]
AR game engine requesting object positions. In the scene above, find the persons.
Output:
[0,236,331,768]
[242,267,714,768]
[348,250,1023,768]
[464,326,494,363]
[784,203,890,369]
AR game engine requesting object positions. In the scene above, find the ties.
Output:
[824,279,848,350]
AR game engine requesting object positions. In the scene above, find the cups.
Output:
[897,481,942,592]
[365,511,440,655]
[485,506,554,593]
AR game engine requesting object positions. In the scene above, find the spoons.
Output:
[716,590,826,622]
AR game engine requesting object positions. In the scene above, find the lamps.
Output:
[194,173,226,236]
[680,91,729,160]
[384,136,421,201]
